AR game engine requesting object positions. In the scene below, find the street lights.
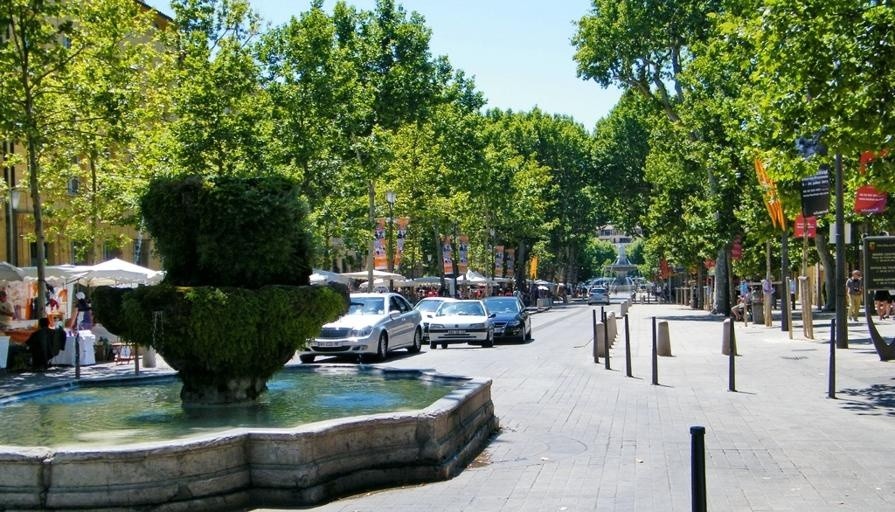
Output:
[9,192,20,267]
[490,228,496,295]
[387,192,396,292]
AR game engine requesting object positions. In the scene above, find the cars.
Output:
[298,293,531,363]
[588,288,610,305]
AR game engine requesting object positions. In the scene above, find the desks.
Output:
[112,342,136,365]
[0,328,96,369]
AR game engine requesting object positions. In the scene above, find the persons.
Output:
[730,278,797,321]
[884,289,895,318]
[847,269,863,321]
[614,286,617,295]
[875,290,891,320]
[656,284,670,303]
[563,288,567,304]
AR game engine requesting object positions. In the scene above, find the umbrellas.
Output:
[309,264,553,301]
[0,256,162,329]
